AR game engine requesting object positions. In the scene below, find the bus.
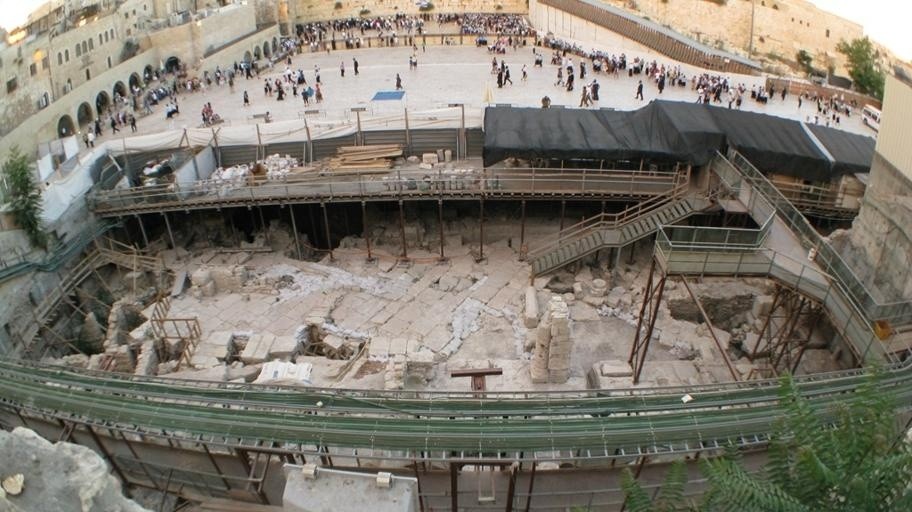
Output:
[860,102,882,130]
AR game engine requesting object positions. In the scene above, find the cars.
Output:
[415,0,426,5]
[419,2,433,11]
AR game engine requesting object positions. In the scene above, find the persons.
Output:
[62,10,852,149]
